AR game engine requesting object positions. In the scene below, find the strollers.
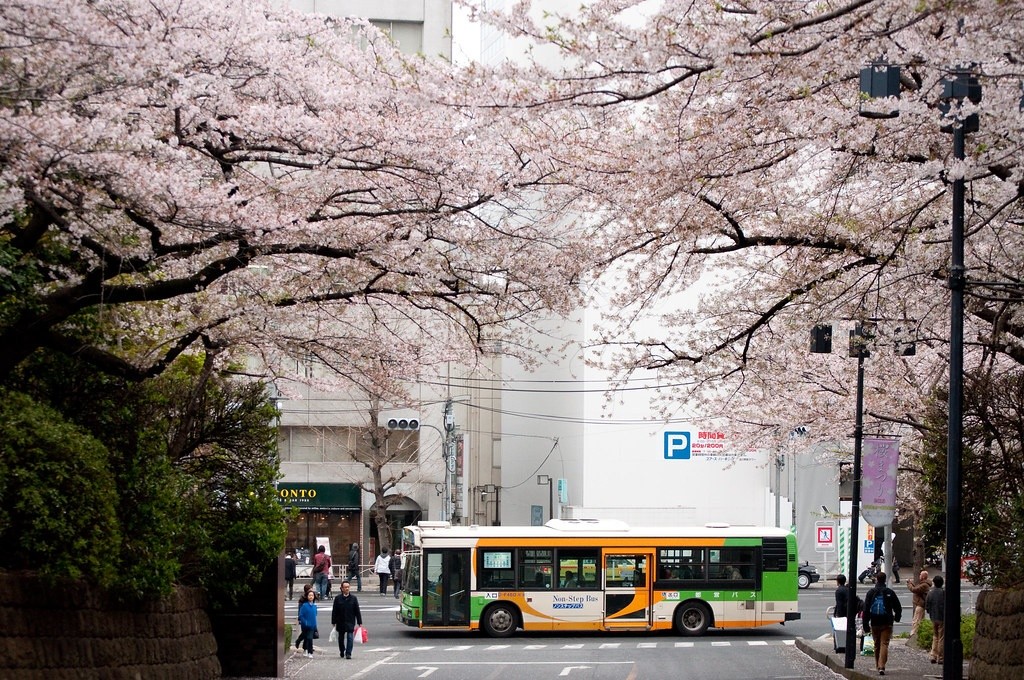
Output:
[858,557,884,584]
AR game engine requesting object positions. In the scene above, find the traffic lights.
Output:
[794,425,812,435]
[385,417,420,430]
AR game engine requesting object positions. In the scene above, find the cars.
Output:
[798,564,820,589]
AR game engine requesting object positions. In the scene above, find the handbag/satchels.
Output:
[329,625,338,642]
[353,626,369,644]
[394,569,402,583]
[854,613,863,638]
[315,555,326,573]
[354,627,363,645]
[313,629,319,639]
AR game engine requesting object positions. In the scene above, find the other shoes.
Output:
[879,670,885,675]
[938,661,944,665]
[284,591,333,601]
[860,651,865,656]
[303,650,308,657]
[295,640,299,649]
[312,649,315,651]
[340,651,344,658]
[931,658,936,664]
[394,595,399,599]
[309,653,314,659]
[358,590,364,593]
[380,593,386,597]
[346,655,351,659]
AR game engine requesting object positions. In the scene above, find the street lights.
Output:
[854,55,986,680]
[484,484,499,526]
[536,474,553,520]
[808,304,917,670]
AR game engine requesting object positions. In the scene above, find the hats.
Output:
[352,543,359,551]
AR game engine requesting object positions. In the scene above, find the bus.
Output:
[396,517,802,640]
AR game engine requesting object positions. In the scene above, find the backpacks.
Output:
[869,587,892,619]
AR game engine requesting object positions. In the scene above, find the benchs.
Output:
[536,571,545,587]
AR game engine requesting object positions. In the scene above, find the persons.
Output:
[311,545,331,600]
[925,576,945,664]
[295,585,318,659]
[834,574,848,617]
[389,549,402,599]
[373,547,391,596]
[906,571,931,638]
[347,543,361,592]
[285,553,296,600]
[331,581,363,659]
[892,556,900,583]
[564,571,576,588]
[862,573,902,675]
